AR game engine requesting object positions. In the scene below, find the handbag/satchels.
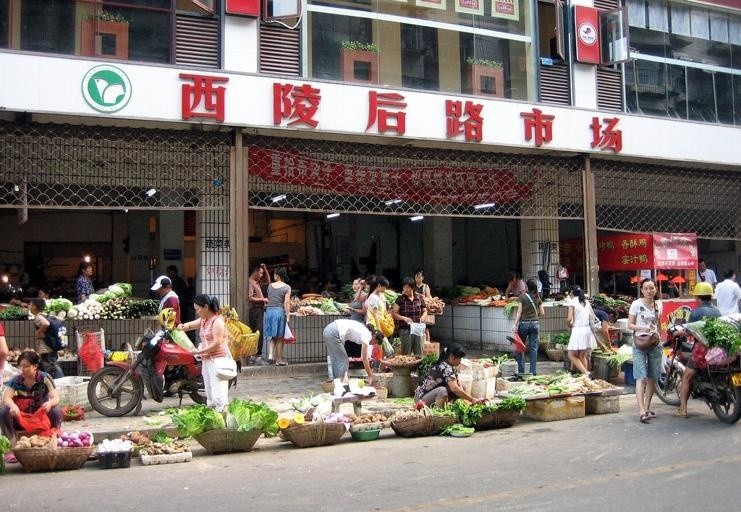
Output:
[13,396,35,414]
[424,314,436,326]
[633,332,660,351]
[211,356,238,379]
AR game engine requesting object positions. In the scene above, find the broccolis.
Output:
[56,309,77,320]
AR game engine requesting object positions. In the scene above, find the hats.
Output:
[150,275,173,291]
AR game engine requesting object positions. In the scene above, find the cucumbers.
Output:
[124,299,161,320]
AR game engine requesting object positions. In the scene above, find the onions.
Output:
[56,430,93,448]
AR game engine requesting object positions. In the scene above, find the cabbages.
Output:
[170,399,280,440]
[90,282,133,303]
[169,328,196,354]
[42,298,73,311]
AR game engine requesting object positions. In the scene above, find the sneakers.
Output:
[266,358,289,366]
[246,355,262,365]
[674,408,689,418]
[639,410,657,422]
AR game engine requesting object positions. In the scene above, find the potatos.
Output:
[145,440,172,455]
[15,434,48,448]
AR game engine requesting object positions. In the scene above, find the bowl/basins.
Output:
[352,430,380,441]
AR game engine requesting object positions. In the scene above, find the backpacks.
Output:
[34,313,69,352]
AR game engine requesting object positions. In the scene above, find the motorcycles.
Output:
[654,312,741,427]
[84,308,241,419]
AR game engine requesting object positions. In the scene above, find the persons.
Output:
[76,260,94,304]
[151,263,230,414]
[0,262,64,462]
[249,261,485,411]
[503,267,617,380]
[714,269,741,315]
[696,257,719,293]
[627,279,664,422]
[671,281,721,417]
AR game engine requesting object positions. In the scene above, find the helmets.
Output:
[691,282,714,296]
[159,307,177,330]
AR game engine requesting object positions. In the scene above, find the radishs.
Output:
[74,299,104,320]
